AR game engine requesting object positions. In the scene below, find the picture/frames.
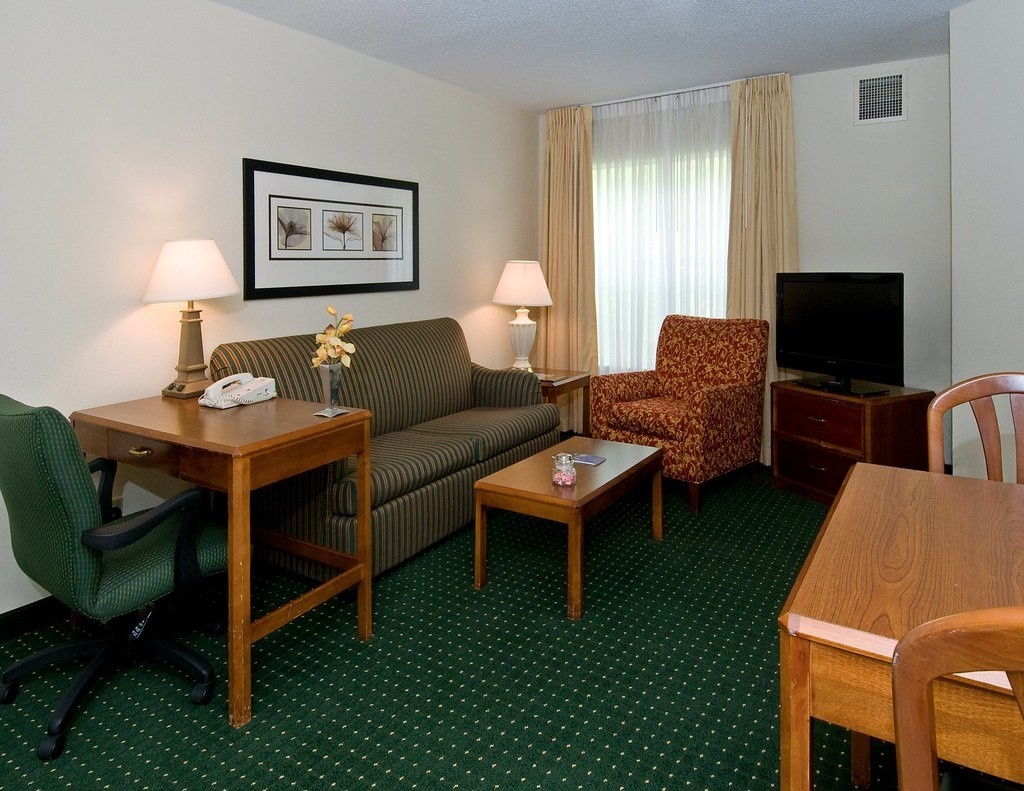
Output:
[241,156,421,302]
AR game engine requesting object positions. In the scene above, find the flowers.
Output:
[308,303,356,367]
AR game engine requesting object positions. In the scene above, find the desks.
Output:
[506,366,592,436]
[775,460,1024,791]
[72,382,374,728]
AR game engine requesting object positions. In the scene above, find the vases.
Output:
[319,362,343,403]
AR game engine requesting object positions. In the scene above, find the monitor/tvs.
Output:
[777,272,904,397]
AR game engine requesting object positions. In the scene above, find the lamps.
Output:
[491,259,555,368]
[142,238,240,395]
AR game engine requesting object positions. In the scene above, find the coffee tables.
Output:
[470,436,665,624]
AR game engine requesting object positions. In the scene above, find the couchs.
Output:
[211,316,560,591]
[590,314,770,516]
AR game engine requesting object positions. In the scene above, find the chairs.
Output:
[926,371,1024,483]
[0,393,229,764]
[887,606,1023,791]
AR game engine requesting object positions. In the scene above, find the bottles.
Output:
[551,452,577,487]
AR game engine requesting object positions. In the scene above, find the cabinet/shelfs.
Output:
[769,376,936,508]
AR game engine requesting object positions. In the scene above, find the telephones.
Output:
[198,373,277,409]
